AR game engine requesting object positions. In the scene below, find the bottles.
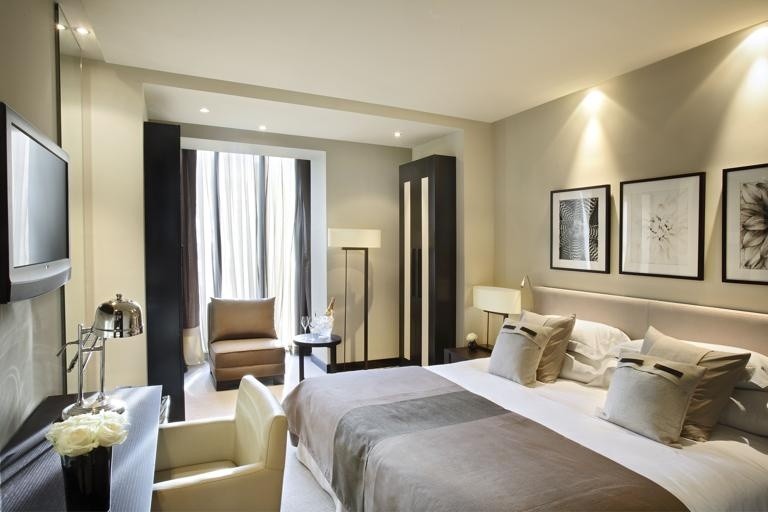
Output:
[324,298,335,316]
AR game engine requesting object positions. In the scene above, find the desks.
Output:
[0,385,162,512]
[293,333,342,382]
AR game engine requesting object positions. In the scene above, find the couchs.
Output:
[207,295,287,391]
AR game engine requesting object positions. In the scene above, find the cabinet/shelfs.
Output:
[398,153,457,372]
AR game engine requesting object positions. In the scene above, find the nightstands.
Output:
[442,345,492,363]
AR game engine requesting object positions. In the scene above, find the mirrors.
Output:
[55,4,98,394]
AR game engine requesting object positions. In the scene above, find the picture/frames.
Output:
[619,170,705,280]
[548,182,612,273]
[722,162,768,286]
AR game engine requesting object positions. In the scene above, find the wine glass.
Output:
[300,315,310,340]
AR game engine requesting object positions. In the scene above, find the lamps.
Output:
[328,228,382,369]
[473,286,522,351]
[55,292,143,421]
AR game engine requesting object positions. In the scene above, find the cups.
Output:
[309,317,335,342]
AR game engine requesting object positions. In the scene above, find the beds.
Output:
[280,276,768,512]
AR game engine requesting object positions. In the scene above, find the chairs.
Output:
[152,373,288,512]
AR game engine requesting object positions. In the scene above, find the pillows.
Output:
[483,308,767,448]
[210,297,278,343]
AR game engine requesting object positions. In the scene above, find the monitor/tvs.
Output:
[0,101,71,306]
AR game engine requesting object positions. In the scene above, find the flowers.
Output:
[39,410,130,457]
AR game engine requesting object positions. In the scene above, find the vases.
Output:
[59,446,111,512]
